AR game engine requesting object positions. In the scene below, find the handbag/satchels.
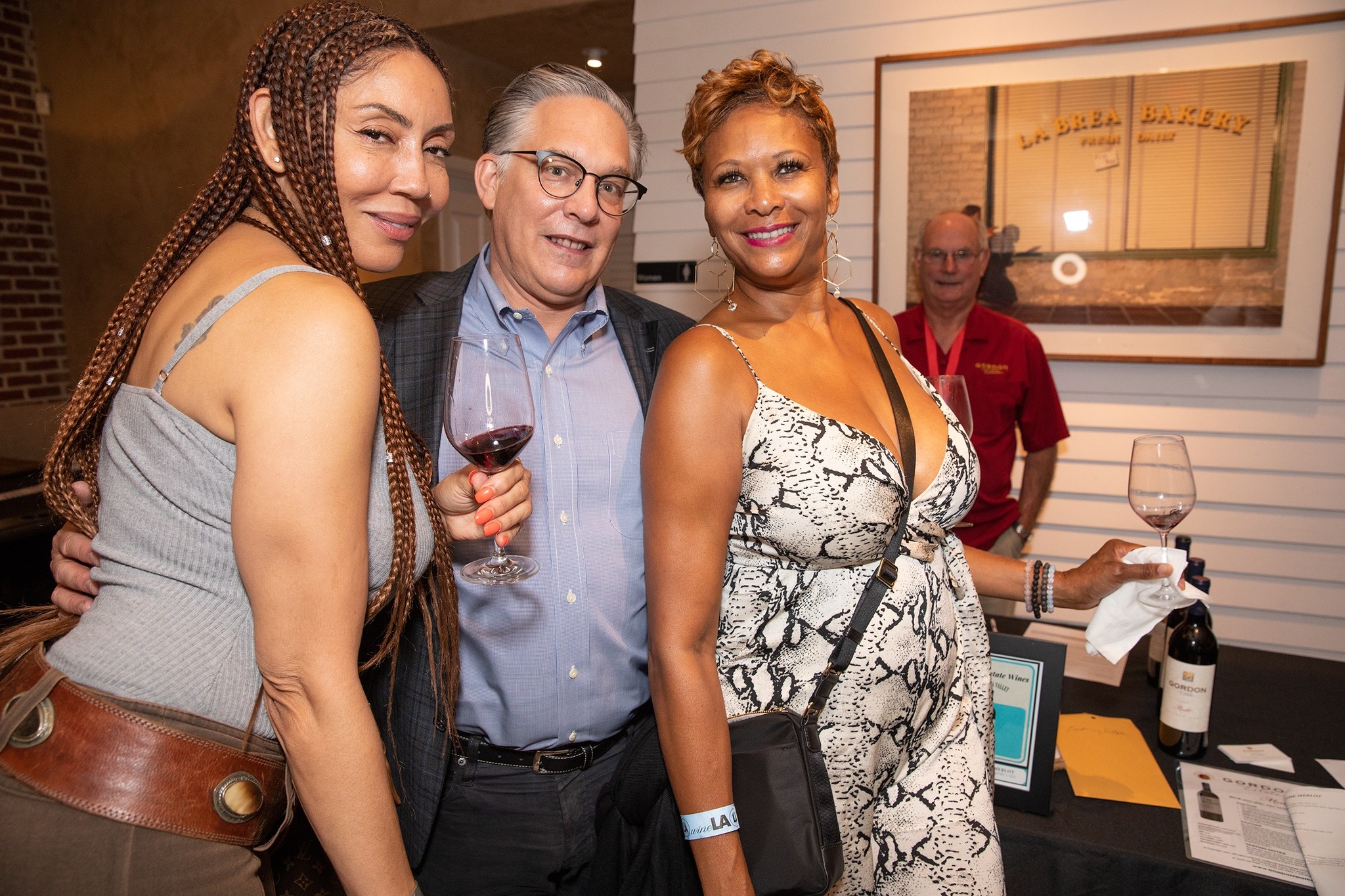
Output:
[728,705,845,896]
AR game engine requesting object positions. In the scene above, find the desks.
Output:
[983,615,1345,896]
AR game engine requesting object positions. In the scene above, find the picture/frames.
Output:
[874,9,1344,368]
[988,633,1068,819]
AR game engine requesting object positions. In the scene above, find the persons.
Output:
[889,210,1071,615]
[642,51,1184,895]
[46,59,706,895]
[0,3,537,896]
[961,204,999,240]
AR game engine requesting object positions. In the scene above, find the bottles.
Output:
[1146,534,1218,759]
[1197,782,1224,822]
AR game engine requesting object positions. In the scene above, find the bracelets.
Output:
[678,804,742,841]
[1023,558,1056,618]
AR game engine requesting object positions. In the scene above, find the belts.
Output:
[450,700,653,775]
[0,640,286,848]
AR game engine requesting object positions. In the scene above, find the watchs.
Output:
[1012,521,1030,538]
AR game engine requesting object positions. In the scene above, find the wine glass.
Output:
[1129,434,1197,608]
[926,374,974,528]
[448,334,540,586]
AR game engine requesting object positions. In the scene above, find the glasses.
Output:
[920,249,982,265]
[495,149,647,217]
